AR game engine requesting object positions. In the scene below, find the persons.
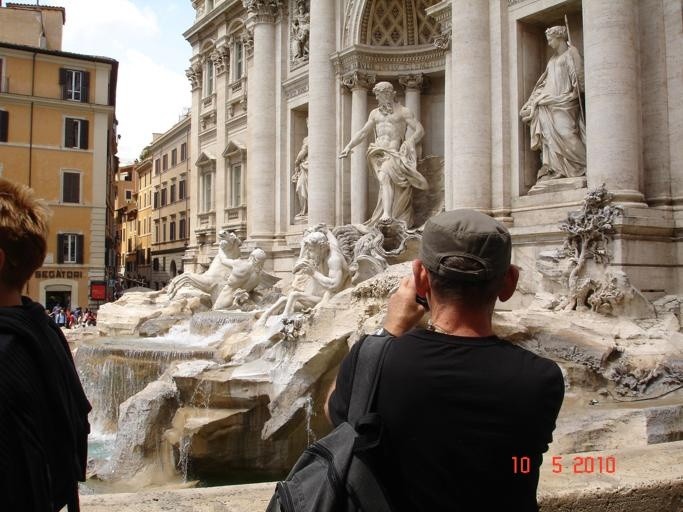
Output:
[290,4,309,59]
[0,174,94,512]
[45,302,96,329]
[519,24,586,180]
[289,116,308,217]
[338,81,431,228]
[278,227,351,316]
[321,206,565,512]
[211,241,266,308]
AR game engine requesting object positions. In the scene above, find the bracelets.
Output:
[373,326,391,337]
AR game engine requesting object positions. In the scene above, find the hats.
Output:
[419,210,511,281]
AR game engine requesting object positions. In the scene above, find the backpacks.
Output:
[267,335,396,511]
